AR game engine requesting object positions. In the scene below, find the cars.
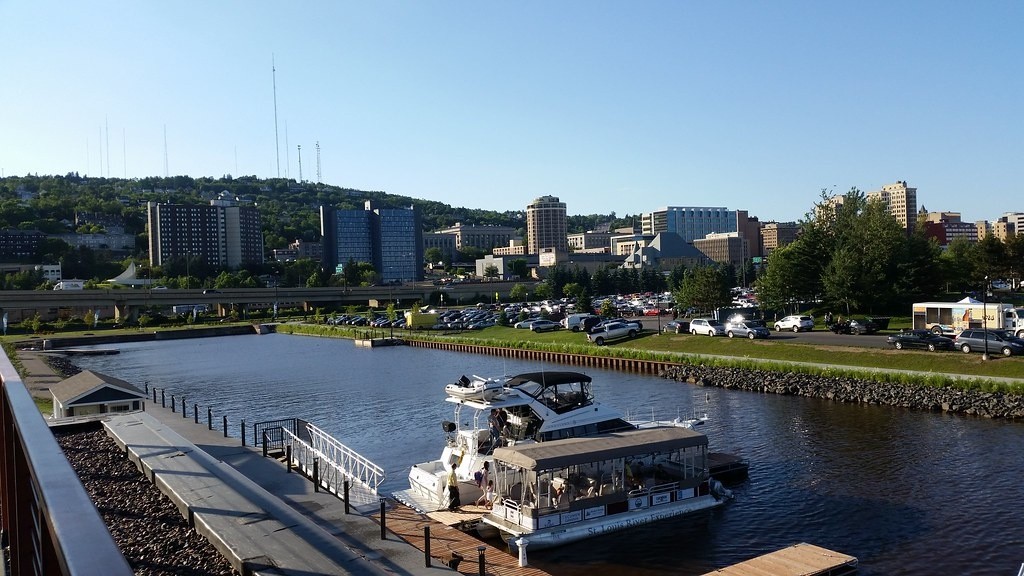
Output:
[887,330,953,351]
[830,318,880,335]
[724,321,769,341]
[728,287,824,307]
[591,318,643,333]
[424,309,501,330]
[663,320,690,334]
[478,291,673,316]
[509,315,518,324]
[326,314,405,328]
[514,319,536,329]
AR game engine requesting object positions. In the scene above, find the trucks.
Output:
[54,281,83,289]
[563,313,594,332]
[406,311,442,331]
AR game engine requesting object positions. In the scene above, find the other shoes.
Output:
[485,505,488,509]
[451,508,460,512]
[475,501,478,508]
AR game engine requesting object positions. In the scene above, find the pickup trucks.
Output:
[587,322,639,346]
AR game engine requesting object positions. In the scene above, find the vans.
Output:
[955,329,1024,357]
[774,315,813,333]
[689,319,726,337]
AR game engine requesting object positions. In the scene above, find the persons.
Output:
[487,408,508,455]
[615,461,669,493]
[835,316,844,335]
[541,472,596,511]
[475,461,494,509]
[824,312,834,329]
[672,303,694,320]
[448,463,460,512]
[810,314,816,329]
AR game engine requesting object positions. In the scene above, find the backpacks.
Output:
[474,471,483,488]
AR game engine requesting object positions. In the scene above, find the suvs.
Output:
[152,286,167,289]
[579,317,603,332]
[530,319,562,333]
[202,289,221,294]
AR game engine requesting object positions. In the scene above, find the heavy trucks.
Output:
[912,301,1024,339]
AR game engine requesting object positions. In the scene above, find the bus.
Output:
[173,304,206,314]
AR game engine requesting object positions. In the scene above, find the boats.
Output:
[408,372,709,508]
[481,426,736,552]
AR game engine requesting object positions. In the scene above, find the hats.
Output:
[491,409,499,412]
